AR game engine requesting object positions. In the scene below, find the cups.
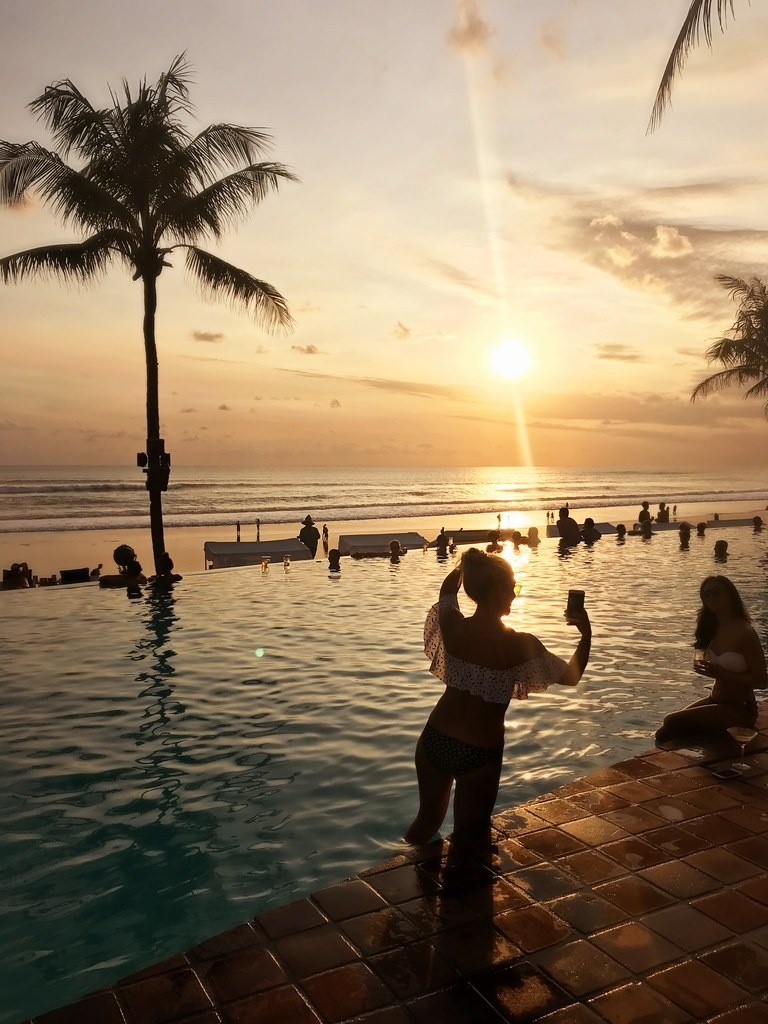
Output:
[693,649,704,667]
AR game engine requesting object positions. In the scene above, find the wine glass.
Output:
[727,727,758,770]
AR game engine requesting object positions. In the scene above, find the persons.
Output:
[0,496,768,589]
[655,573,768,755]
[406,550,590,868]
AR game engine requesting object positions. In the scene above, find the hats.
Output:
[301,515,315,525]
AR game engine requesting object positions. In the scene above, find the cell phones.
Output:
[568,590,585,622]
